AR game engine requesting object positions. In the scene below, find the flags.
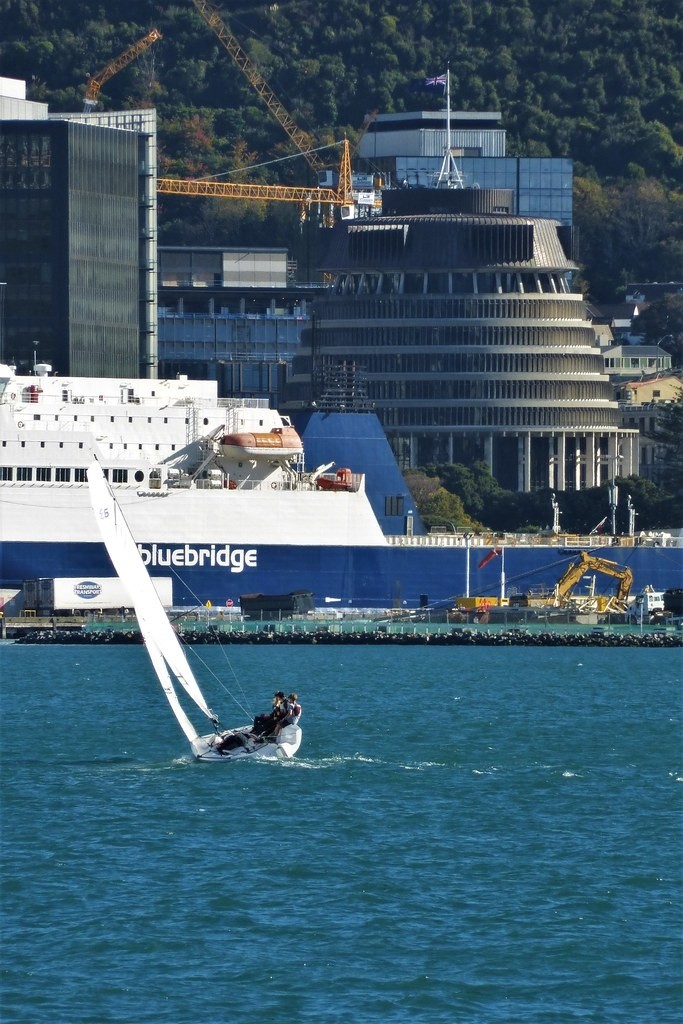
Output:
[425,74,446,86]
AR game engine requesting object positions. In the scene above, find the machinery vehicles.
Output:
[509,550,633,612]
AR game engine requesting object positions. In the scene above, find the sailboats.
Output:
[86,454,304,763]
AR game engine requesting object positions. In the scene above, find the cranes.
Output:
[81,0,355,228]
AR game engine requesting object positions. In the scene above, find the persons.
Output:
[253,691,302,743]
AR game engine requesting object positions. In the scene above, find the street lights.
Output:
[463,531,476,598]
